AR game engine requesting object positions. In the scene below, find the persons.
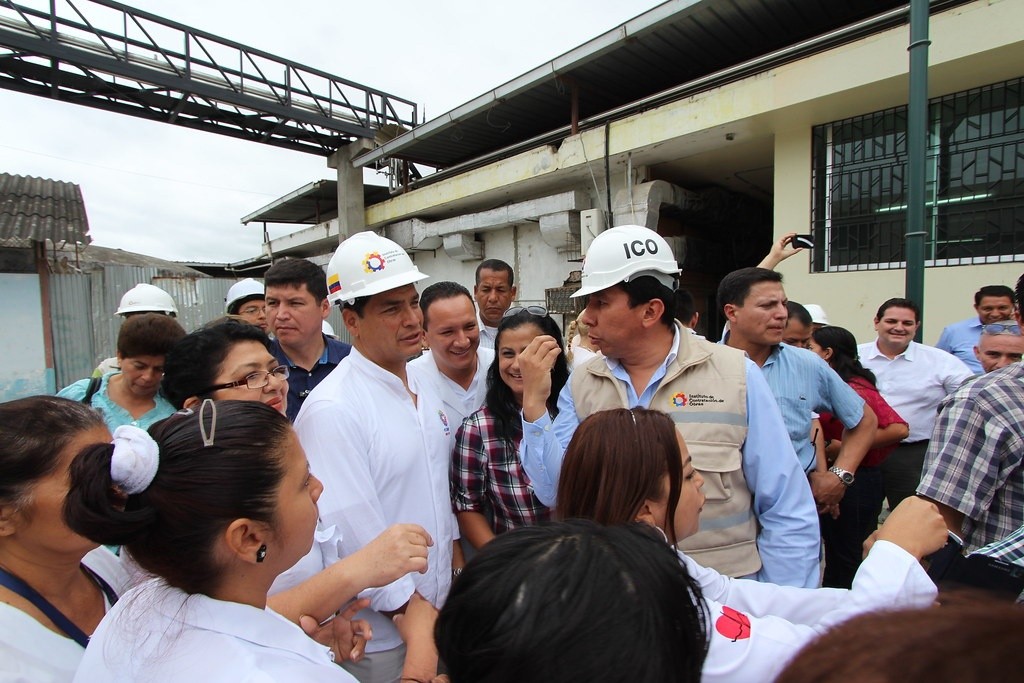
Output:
[293,230,464,683]
[518,225,823,589]
[431,519,715,683]
[0,231,1024,683]
[554,407,949,683]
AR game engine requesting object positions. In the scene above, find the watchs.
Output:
[829,466,858,488]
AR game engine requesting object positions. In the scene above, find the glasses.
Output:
[195,364,291,398]
[978,324,1023,336]
[501,305,549,319]
[239,306,266,315]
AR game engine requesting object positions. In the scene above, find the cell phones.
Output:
[791,235,814,249]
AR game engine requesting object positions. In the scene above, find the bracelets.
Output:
[452,568,463,576]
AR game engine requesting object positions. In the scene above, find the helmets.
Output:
[113,283,179,318]
[568,225,680,297]
[325,230,428,305]
[804,303,828,325]
[225,277,266,312]
